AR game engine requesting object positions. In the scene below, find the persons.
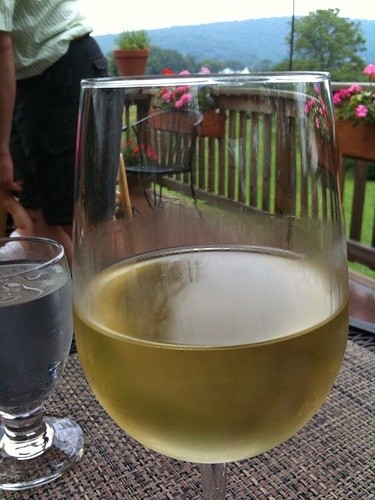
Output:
[0,0,124,259]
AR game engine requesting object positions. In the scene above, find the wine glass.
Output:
[71,70,349,500]
[0,235,85,491]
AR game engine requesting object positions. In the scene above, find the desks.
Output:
[0,316,375,500]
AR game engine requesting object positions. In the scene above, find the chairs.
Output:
[122,108,203,209]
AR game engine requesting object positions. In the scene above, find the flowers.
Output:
[153,67,219,112]
[302,62,375,128]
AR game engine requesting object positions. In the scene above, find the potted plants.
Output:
[113,27,151,76]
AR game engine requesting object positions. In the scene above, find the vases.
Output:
[335,122,375,160]
[149,109,227,139]
